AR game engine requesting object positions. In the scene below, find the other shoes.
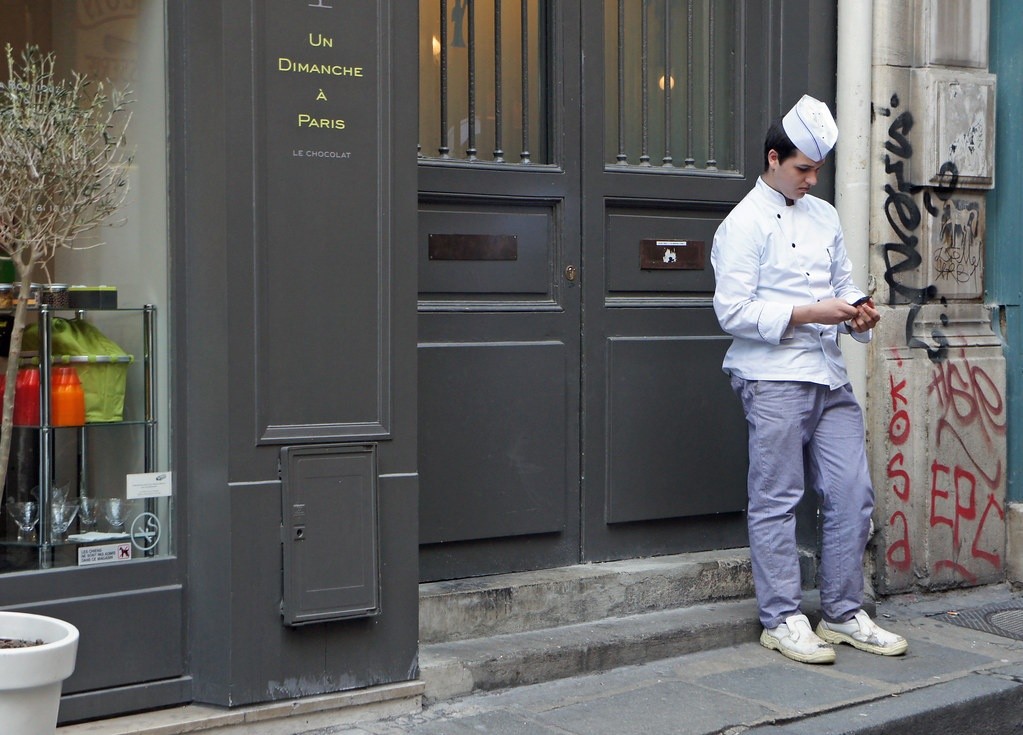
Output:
[814,609,909,656]
[758,614,836,663]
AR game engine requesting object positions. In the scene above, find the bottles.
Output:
[51,368,85,426]
[0,369,39,426]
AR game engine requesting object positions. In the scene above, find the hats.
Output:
[782,93,838,163]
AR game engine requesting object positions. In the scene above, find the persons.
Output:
[710,94,910,664]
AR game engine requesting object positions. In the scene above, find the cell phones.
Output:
[851,296,872,308]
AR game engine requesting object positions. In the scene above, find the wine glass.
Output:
[76,496,103,536]
[6,501,39,542]
[51,502,81,543]
[99,498,136,533]
